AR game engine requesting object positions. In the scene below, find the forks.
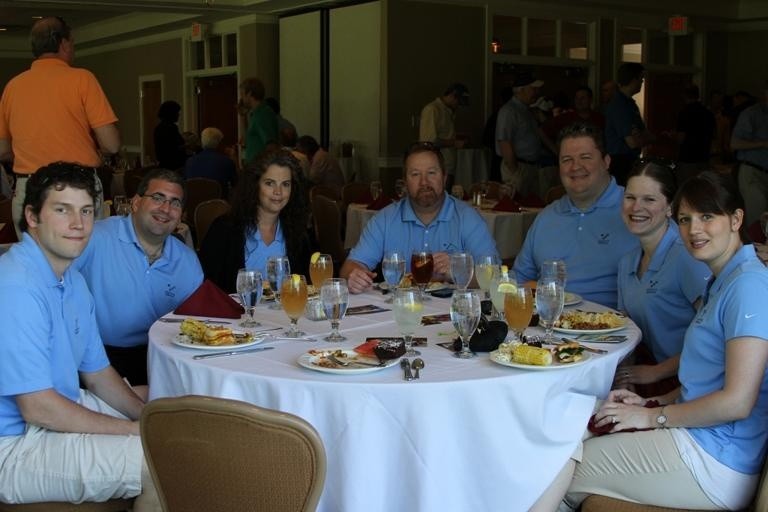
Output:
[325,355,387,368]
[252,333,317,344]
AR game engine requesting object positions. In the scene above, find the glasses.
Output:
[40,161,95,185]
[143,192,182,209]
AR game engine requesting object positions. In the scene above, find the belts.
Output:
[516,158,536,165]
[739,160,768,174]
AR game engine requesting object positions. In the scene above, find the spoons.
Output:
[411,358,425,379]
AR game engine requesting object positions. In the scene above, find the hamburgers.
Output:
[204,325,235,346]
[523,281,539,303]
[555,343,582,363]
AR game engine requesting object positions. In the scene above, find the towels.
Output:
[173,279,245,319]
[746,220,767,242]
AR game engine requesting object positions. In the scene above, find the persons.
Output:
[525,165,768,511]
[70,166,207,391]
[338,140,502,295]
[0,14,122,244]
[195,144,339,297]
[0,159,165,511]
[506,125,643,312]
[152,58,767,226]
[611,155,715,400]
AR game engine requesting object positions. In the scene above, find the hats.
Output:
[448,83,472,97]
[511,72,545,89]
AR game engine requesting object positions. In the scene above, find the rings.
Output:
[624,373,629,375]
[611,415,617,424]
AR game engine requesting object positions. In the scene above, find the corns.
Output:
[511,345,552,365]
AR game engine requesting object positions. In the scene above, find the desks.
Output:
[111,168,126,199]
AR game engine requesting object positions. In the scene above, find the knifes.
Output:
[561,337,607,354]
[158,317,232,325]
[400,358,412,382]
[193,346,274,360]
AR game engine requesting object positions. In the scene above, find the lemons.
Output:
[498,283,516,293]
[290,273,300,286]
[310,253,320,263]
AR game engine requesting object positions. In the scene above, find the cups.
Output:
[471,188,485,209]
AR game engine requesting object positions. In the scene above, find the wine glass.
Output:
[448,250,533,345]
[318,276,349,343]
[451,184,463,200]
[449,290,482,360]
[113,195,135,216]
[309,254,332,299]
[96,149,136,175]
[393,287,423,358]
[396,178,406,198]
[541,256,567,290]
[265,256,290,310]
[279,274,308,337]
[235,268,264,328]
[410,250,433,302]
[369,180,384,202]
[381,250,406,304]
[534,278,564,342]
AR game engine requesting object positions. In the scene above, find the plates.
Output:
[489,345,591,370]
[531,288,582,307]
[296,347,400,375]
[378,280,448,292]
[170,328,265,351]
[538,312,628,334]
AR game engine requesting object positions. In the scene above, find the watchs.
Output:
[656,405,667,429]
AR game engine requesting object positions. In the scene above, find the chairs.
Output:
[581,464,768,512]
[0,199,14,244]
[104,167,113,201]
[0,496,136,511]
[139,395,326,512]
